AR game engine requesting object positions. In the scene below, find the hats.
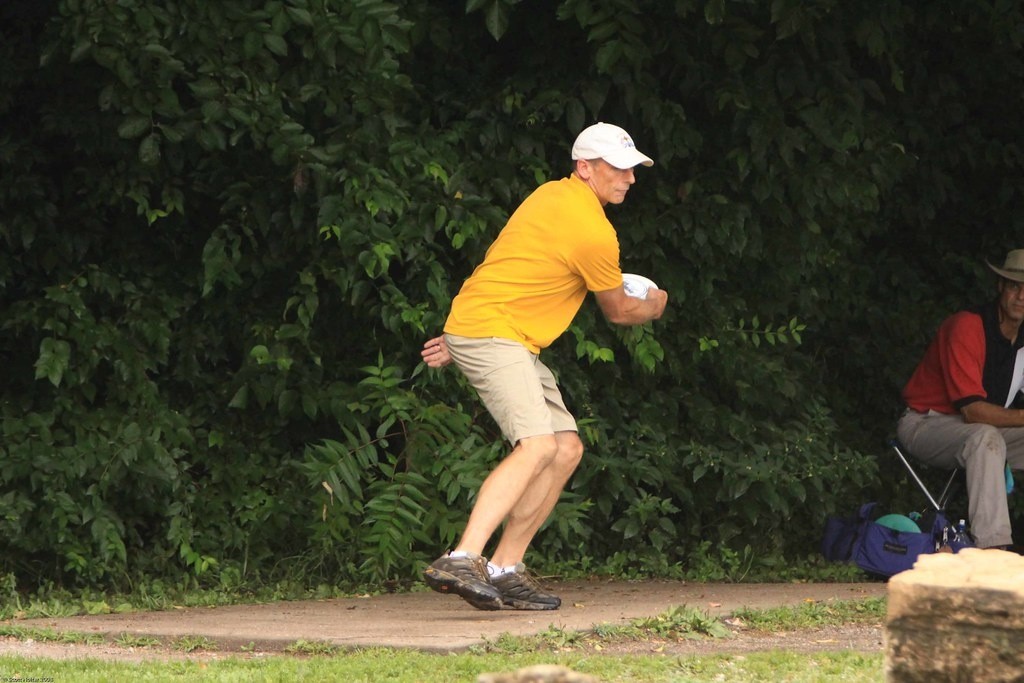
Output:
[985,249,1024,282]
[571,122,654,169]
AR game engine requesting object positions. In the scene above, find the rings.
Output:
[438,346,441,351]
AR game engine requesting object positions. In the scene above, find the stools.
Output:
[886,433,1013,549]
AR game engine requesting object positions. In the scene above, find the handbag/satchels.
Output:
[820,497,949,578]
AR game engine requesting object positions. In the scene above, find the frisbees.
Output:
[874,514,922,533]
[621,273,658,300]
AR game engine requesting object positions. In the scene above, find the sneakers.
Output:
[423,549,503,610]
[488,563,561,610]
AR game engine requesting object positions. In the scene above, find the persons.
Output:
[897,248,1024,551]
[421,121,668,609]
